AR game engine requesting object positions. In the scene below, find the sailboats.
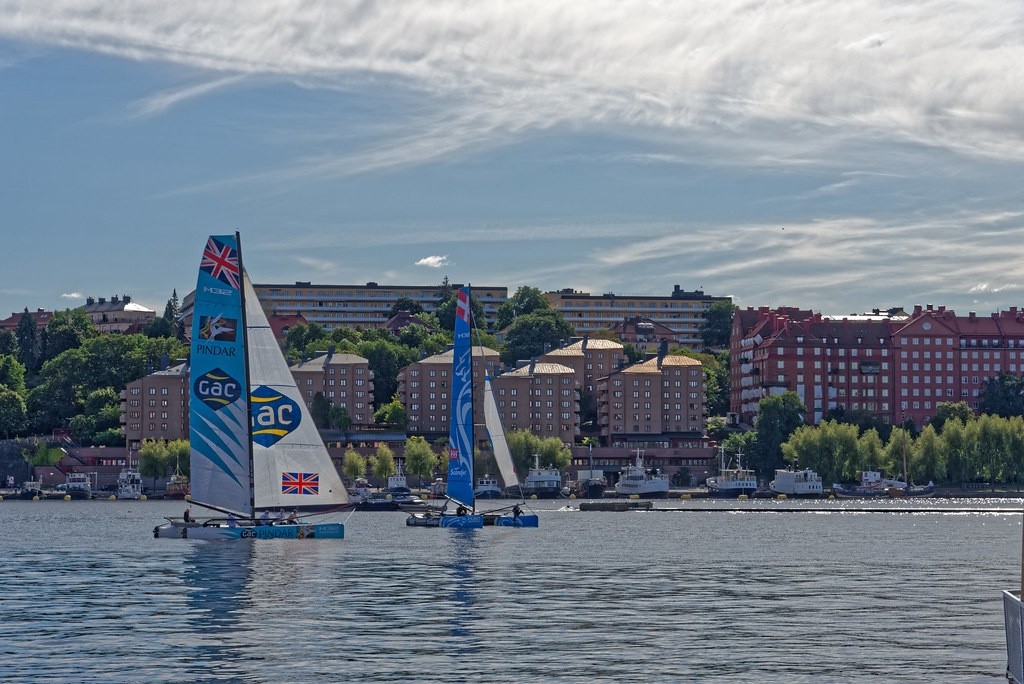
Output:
[152,229,353,540]
[407,281,540,528]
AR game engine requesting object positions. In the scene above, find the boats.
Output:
[575,442,607,499]
[347,458,449,512]
[427,477,448,499]
[520,449,561,498]
[706,442,758,497]
[2,454,190,501]
[769,455,823,499]
[834,474,937,500]
[615,446,670,499]
[473,472,501,499]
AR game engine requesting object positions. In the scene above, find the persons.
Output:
[183,508,194,522]
[275,509,286,525]
[456,504,467,516]
[258,510,270,525]
[286,510,301,525]
[226,513,241,528]
[512,505,525,523]
